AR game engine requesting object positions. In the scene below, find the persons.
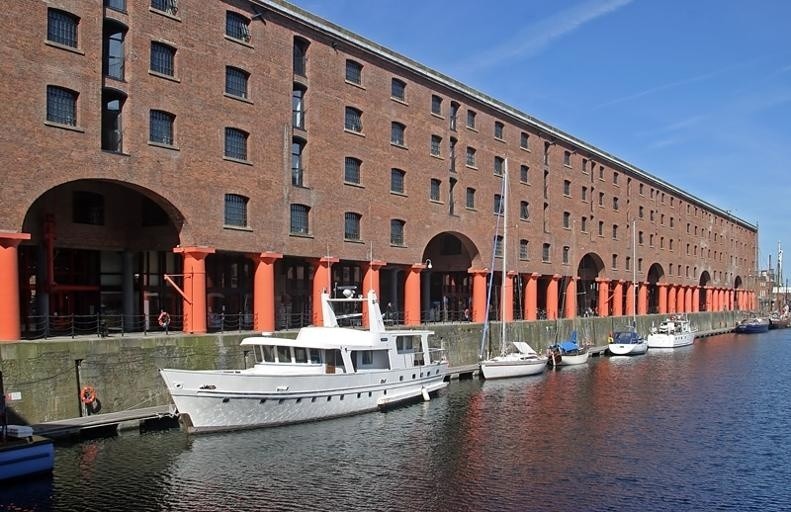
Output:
[463,306,470,320]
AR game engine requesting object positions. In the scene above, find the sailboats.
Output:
[732,259,791,333]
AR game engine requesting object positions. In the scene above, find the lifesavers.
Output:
[159,312,171,328]
[81,387,96,404]
[465,309,470,319]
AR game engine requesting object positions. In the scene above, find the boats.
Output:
[158,240,451,433]
[479,158,650,379]
[647,311,697,349]
